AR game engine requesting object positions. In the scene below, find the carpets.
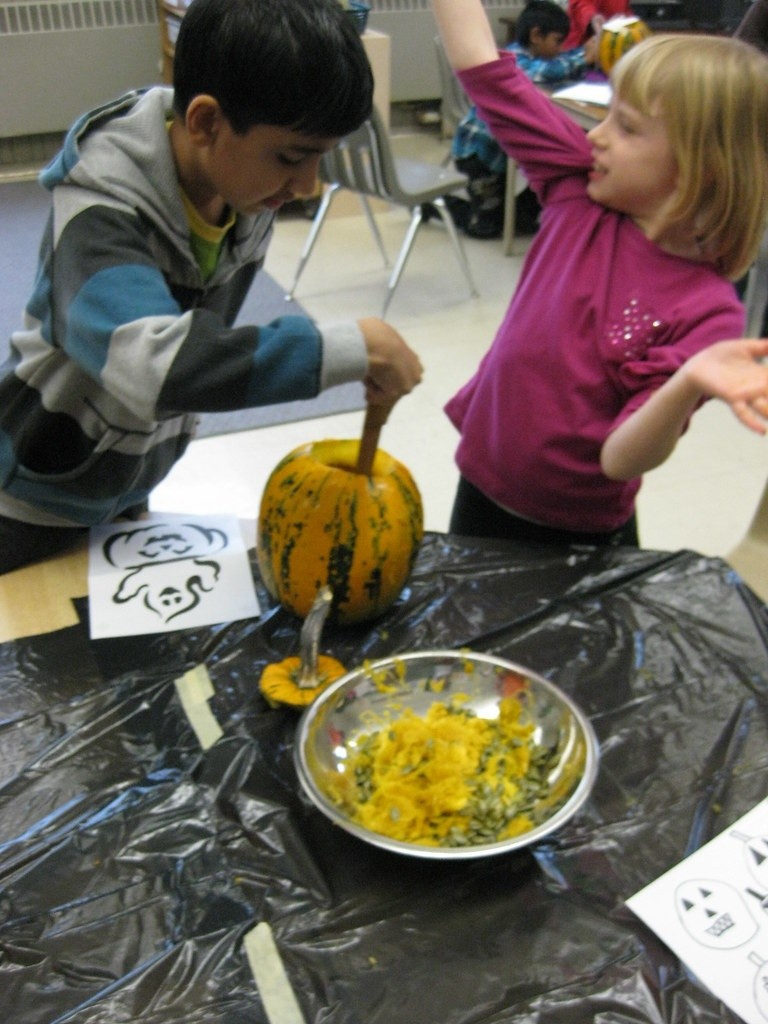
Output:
[0,179,368,438]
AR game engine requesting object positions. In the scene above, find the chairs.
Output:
[283,106,478,316]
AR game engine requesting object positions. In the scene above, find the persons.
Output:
[421,0,593,239]
[432,0,768,548]
[0,0,426,579]
[558,0,633,53]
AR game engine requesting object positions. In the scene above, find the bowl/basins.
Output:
[292,649,598,860]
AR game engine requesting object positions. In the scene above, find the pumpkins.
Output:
[258,584,349,708]
[599,17,652,75]
[259,438,424,625]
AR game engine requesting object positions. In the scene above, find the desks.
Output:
[502,86,609,256]
[0,513,768,1024]
